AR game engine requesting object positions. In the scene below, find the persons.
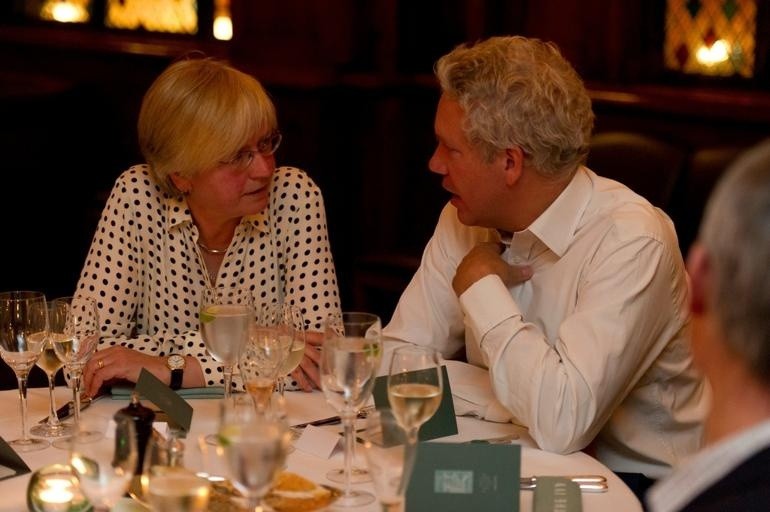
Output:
[64,57,345,396]
[367,34,713,498]
[639,140,769,510]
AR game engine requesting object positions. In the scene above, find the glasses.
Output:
[219,129,283,175]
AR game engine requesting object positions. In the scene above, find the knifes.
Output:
[37,393,109,425]
[290,410,368,429]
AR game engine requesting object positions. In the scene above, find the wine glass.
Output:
[238,306,294,418]
[1,294,50,453]
[258,306,303,414]
[197,287,257,403]
[25,303,75,439]
[387,345,444,446]
[56,295,100,453]
[318,310,383,508]
[29,414,330,511]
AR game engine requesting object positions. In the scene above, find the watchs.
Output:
[164,352,187,390]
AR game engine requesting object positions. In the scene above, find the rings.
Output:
[97,357,105,368]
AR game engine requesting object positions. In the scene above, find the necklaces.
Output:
[196,240,229,256]
